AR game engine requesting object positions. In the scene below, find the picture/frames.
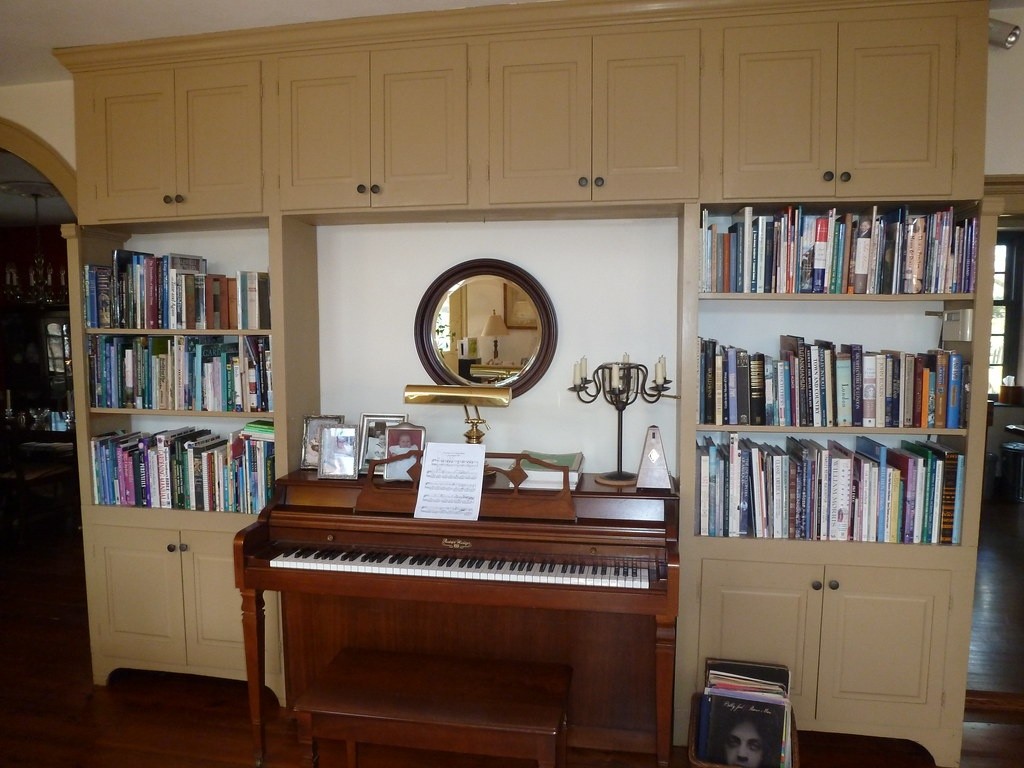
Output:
[359,412,409,477]
[385,423,426,482]
[316,423,359,480]
[503,282,538,331]
[300,415,347,470]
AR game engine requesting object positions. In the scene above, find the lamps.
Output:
[0,179,69,311]
[405,385,513,484]
[481,309,509,366]
[470,364,524,383]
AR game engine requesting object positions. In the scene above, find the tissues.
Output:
[999,375,1023,404]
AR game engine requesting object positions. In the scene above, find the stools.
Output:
[1002,441,1024,501]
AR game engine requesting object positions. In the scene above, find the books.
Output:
[694,432,965,545]
[457,337,477,358]
[698,658,792,768]
[509,450,584,490]
[88,332,273,412]
[83,249,270,329]
[90,420,275,514]
[697,335,970,429]
[699,206,978,295]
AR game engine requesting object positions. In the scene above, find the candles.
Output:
[658,355,667,377]
[611,362,620,388]
[6,388,12,410]
[623,352,630,363]
[574,362,581,386]
[581,356,588,379]
[655,361,664,384]
[66,390,73,412]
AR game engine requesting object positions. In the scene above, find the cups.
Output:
[30,409,49,420]
[25,284,66,304]
[67,410,74,422]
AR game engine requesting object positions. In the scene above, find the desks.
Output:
[0,412,74,442]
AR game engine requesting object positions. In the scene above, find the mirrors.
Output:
[413,257,558,402]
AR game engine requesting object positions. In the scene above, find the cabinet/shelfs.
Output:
[262,8,477,213]
[50,28,263,229]
[707,1,988,203]
[479,1,704,210]
[61,216,320,709]
[671,203,997,768]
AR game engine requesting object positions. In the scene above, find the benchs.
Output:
[295,640,575,768]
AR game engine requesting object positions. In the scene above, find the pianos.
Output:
[230,469,681,767]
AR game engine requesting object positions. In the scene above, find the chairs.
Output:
[0,416,72,545]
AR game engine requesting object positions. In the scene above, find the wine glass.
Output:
[1,285,26,303]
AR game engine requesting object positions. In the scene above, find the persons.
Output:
[860,221,870,237]
[306,436,349,472]
[389,434,418,479]
[714,704,779,768]
[374,431,386,459]
